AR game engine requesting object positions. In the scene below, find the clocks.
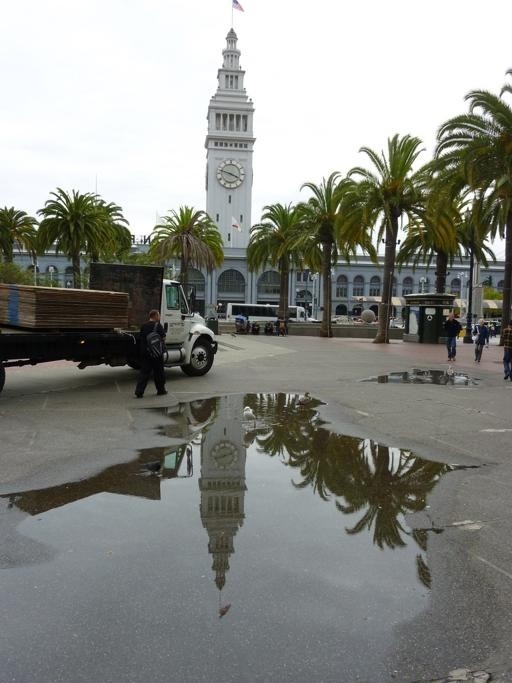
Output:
[209,441,238,469]
[216,159,246,188]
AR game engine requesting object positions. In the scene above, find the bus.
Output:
[224,303,308,324]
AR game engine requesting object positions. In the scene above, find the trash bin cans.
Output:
[235,315,246,333]
[207,317,218,334]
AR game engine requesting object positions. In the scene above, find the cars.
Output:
[331,314,401,324]
[481,317,503,326]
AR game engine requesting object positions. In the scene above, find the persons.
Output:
[490,322,496,338]
[443,312,460,360]
[499,319,511,381]
[472,319,488,361]
[135,309,168,397]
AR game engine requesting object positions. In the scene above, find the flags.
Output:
[219,603,232,617]
[231,216,241,232]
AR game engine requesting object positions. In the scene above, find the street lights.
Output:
[381,237,402,245]
[420,275,427,292]
[456,270,468,316]
[309,270,319,318]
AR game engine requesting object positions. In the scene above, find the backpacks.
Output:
[146,322,163,361]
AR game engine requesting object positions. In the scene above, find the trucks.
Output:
[0,261,222,389]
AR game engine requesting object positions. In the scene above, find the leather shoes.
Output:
[157,389,168,395]
[135,391,143,398]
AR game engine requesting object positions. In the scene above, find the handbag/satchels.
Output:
[471,335,479,341]
